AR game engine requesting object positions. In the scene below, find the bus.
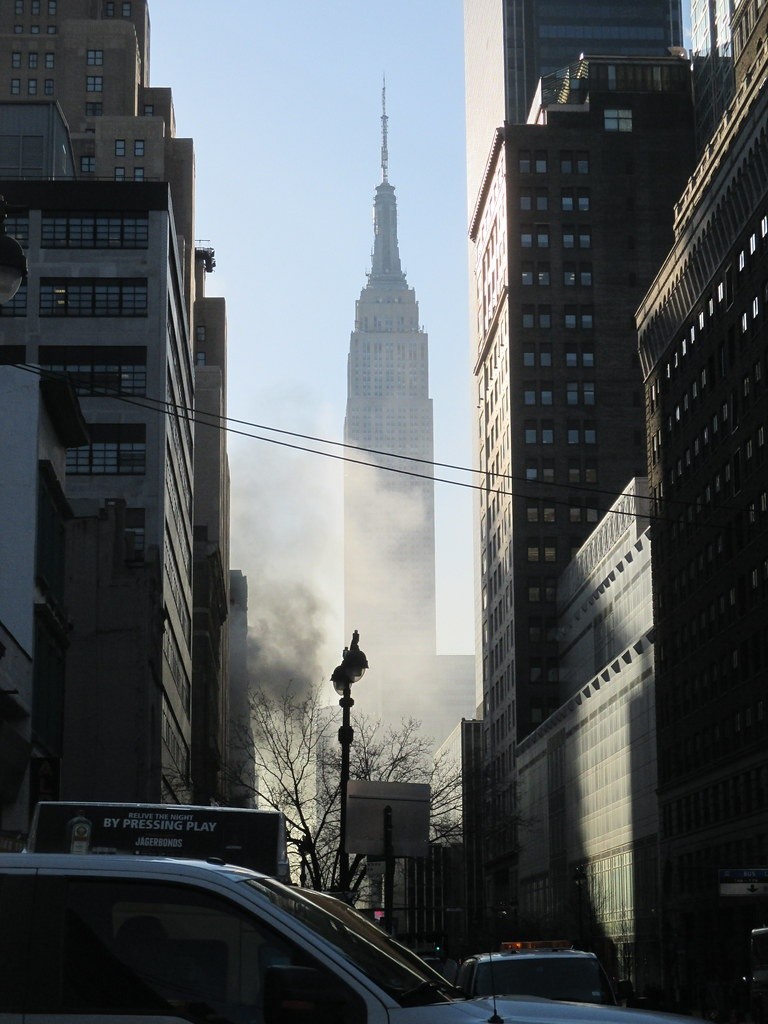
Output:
[26,800,292,885]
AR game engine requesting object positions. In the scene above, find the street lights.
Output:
[329,630,370,891]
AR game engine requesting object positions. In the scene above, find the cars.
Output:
[455,940,618,1007]
[0,849,714,1023]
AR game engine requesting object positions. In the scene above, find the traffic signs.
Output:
[719,868,768,896]
